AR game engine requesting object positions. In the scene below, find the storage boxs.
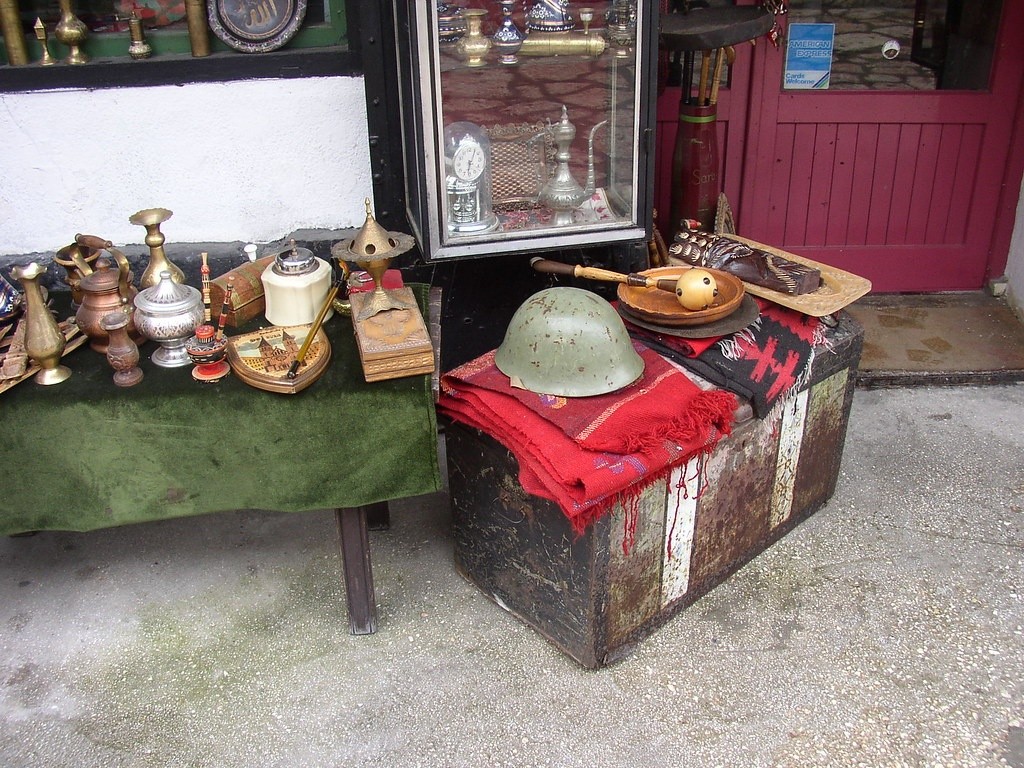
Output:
[441,292,865,670]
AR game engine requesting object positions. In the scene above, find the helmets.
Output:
[494,287,646,397]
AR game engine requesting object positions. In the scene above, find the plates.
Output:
[616,293,761,337]
[617,265,746,325]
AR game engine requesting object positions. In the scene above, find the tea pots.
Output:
[527,106,609,226]
[68,232,148,353]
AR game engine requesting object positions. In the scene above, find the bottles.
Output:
[665,100,720,243]
[127,11,153,61]
[262,238,334,327]
[129,209,186,288]
[101,313,144,387]
[492,0,522,64]
[605,1,637,60]
[8,263,71,387]
[55,1,91,65]
[456,8,492,67]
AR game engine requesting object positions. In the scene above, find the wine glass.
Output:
[578,7,594,35]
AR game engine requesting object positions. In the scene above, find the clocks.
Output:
[444,138,486,183]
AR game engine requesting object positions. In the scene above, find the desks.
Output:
[0,282,443,634]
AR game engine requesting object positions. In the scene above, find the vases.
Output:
[129,208,185,290]
[435,0,467,43]
[9,262,72,386]
[455,8,493,68]
[491,0,523,65]
[53,242,103,314]
[528,0,576,31]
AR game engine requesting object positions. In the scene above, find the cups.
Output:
[54,243,103,313]
